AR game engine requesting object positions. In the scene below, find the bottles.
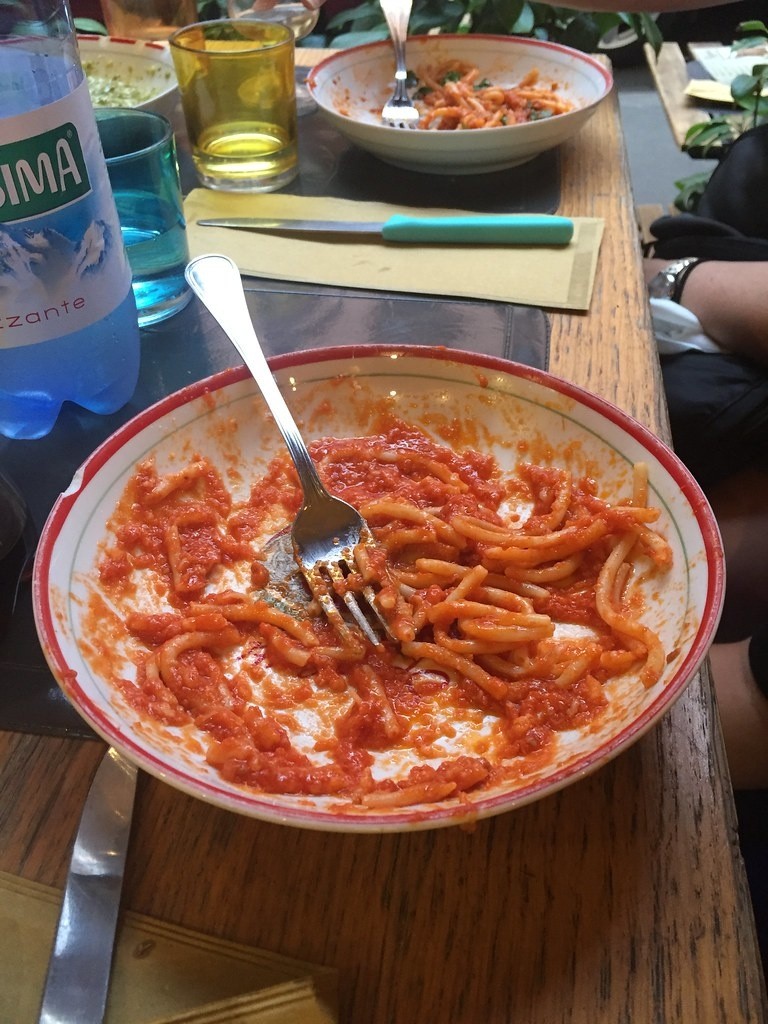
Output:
[0,0,142,441]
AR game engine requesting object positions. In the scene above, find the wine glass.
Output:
[227,0,320,117]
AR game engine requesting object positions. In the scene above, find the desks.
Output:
[645,41,768,152]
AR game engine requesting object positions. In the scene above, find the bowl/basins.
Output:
[307,34,614,175]
[31,343,725,834]
[74,34,196,118]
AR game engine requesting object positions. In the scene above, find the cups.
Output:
[169,18,296,194]
[90,109,194,328]
[101,1,205,53]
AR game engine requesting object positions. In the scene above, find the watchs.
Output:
[648,255,699,301]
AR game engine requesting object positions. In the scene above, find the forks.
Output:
[183,253,402,650]
[379,0,419,132]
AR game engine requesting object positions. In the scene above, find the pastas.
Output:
[414,60,570,129]
[140,461,675,809]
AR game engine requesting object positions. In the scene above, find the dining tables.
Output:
[1,42,767,1022]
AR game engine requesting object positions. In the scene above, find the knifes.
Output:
[197,215,573,244]
[37,749,142,1024]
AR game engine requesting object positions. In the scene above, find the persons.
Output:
[250,0,768,789]
